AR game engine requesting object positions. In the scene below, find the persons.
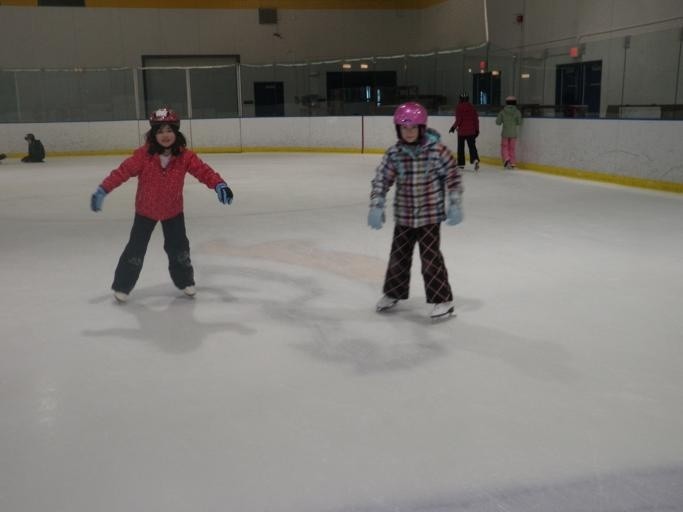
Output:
[447,90,480,170]
[366,102,462,319]
[0,151,6,162]
[90,106,232,303]
[20,133,45,163]
[492,94,523,169]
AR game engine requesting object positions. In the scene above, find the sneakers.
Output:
[474,159,479,169]
[429,302,455,318]
[510,164,516,167]
[376,296,395,307]
[456,164,464,169]
[115,292,128,303]
[184,286,196,296]
[504,159,509,168]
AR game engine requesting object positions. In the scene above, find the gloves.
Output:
[91,186,107,212]
[367,198,385,229]
[215,182,233,204]
[446,197,463,225]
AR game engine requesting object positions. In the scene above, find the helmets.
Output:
[394,103,428,139]
[149,108,180,126]
[460,91,470,98]
[25,133,34,139]
[505,95,516,101]
